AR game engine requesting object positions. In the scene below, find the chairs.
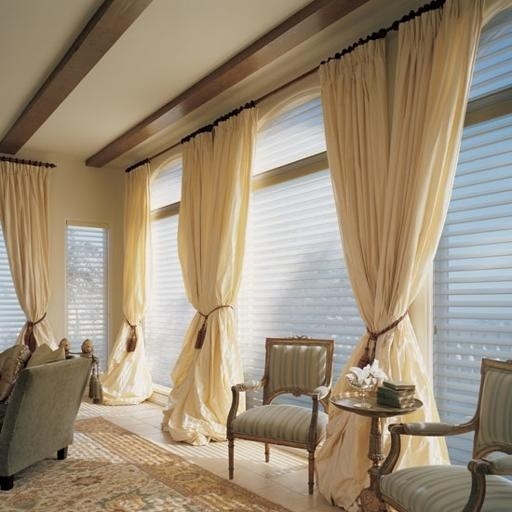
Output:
[0,354,98,489]
[375,357,512,512]
[226,335,334,495]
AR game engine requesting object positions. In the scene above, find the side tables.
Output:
[330,392,423,512]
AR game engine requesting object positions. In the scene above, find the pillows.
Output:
[0,343,67,408]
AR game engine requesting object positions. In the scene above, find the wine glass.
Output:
[346,378,378,408]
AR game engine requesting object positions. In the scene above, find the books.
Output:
[383,379,415,390]
[376,396,415,409]
[376,385,415,400]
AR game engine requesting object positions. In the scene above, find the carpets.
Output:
[0,416,294,512]
